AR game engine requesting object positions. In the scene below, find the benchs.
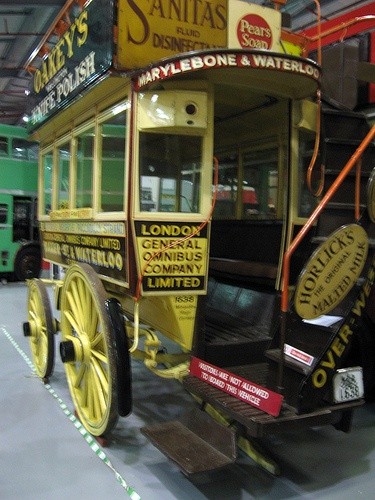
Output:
[208,219,313,290]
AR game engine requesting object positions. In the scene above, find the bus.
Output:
[0,124,128,280]
